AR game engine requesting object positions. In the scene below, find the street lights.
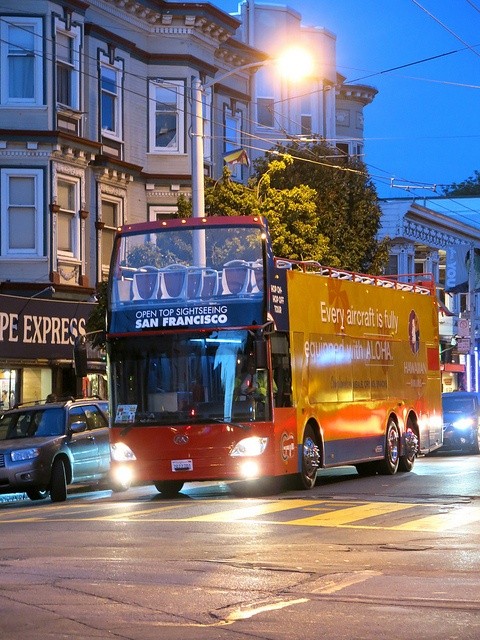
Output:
[189,43,315,265]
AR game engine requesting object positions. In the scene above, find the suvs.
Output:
[0,394,108,501]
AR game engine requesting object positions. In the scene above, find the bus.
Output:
[106,213,444,494]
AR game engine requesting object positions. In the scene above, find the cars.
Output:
[426,392,480,456]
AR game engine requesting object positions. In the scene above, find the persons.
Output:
[233,359,278,410]
